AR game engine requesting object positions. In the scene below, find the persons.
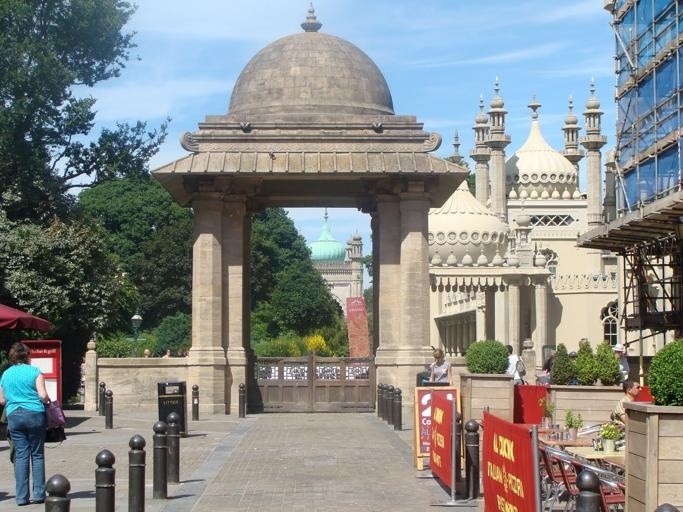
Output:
[568,352,580,386]
[144,349,151,358]
[613,343,630,386]
[163,350,171,358]
[615,381,642,428]
[543,351,556,371]
[506,344,525,385]
[0,341,52,507]
[429,349,453,386]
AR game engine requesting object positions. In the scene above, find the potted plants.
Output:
[622,336,683,512]
[458,338,515,424]
[539,339,628,451]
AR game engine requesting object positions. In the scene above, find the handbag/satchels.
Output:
[516,360,526,376]
[44,401,67,443]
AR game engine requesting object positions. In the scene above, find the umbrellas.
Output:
[0,302,51,331]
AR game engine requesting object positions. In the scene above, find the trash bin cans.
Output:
[157,380,187,438]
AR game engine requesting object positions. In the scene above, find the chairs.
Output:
[538,447,625,512]
